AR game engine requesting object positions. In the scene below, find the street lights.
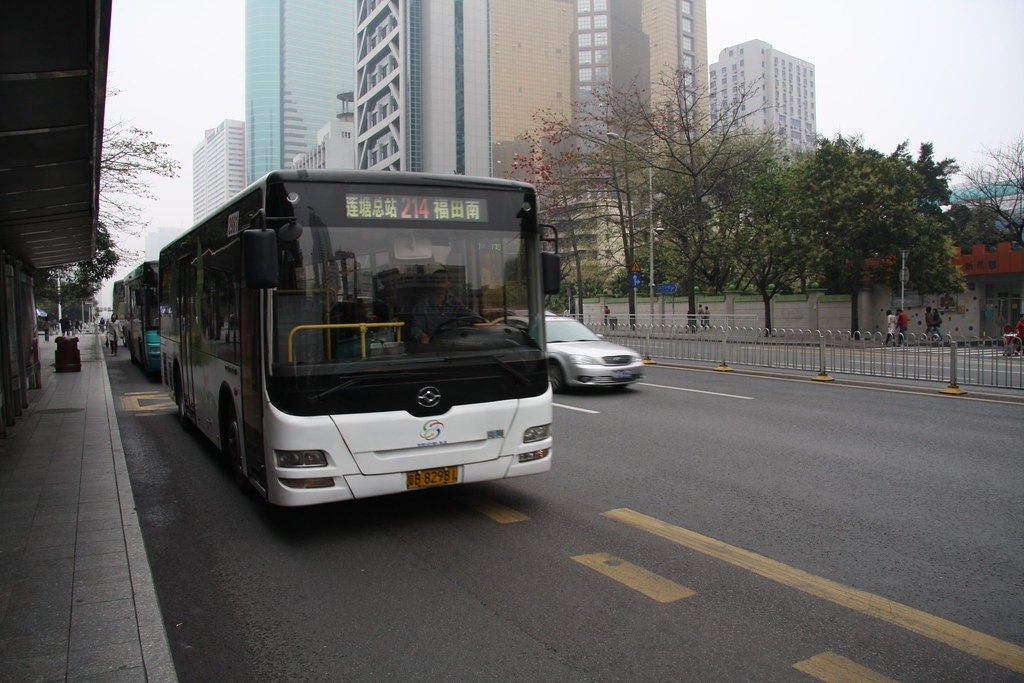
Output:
[607,131,665,324]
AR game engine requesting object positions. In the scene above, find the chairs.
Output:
[388,272,428,342]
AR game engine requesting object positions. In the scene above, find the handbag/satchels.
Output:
[106,340,108,348]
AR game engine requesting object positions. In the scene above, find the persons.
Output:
[895,308,908,347]
[1014,313,1024,356]
[59,315,81,336]
[564,307,570,317]
[885,310,896,347]
[408,270,475,346]
[698,304,704,324]
[107,313,123,356]
[100,317,109,330]
[703,306,711,330]
[604,305,610,325]
[43,318,51,341]
[924,306,943,341]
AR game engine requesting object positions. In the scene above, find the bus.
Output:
[113,169,561,508]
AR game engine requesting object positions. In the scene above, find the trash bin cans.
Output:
[609,318,617,330]
[1003,332,1018,356]
[54,336,82,372]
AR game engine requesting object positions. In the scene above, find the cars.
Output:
[545,316,646,393]
